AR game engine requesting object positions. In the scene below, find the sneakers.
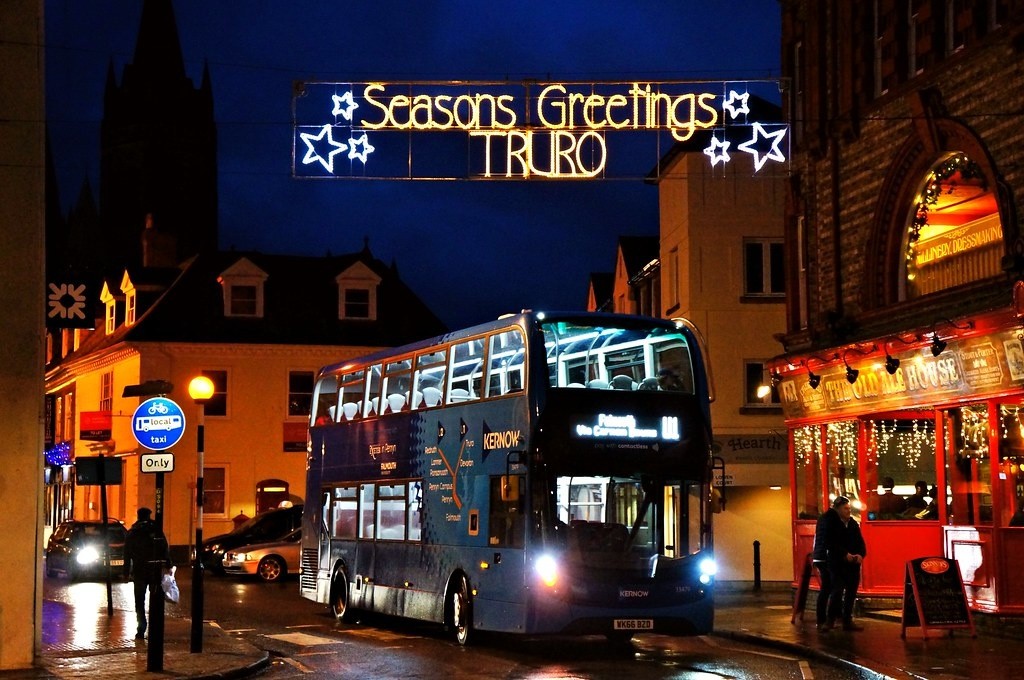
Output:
[135,632,144,639]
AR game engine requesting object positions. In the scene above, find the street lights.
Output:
[188,375,216,655]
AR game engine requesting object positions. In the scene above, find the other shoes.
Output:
[815,623,832,634]
[821,621,836,631]
[842,617,865,632]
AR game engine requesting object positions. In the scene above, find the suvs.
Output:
[190,501,303,577]
[47,515,133,582]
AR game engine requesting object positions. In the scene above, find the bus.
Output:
[297,305,721,651]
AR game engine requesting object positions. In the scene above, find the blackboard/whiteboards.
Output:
[907,556,975,629]
[793,553,813,615]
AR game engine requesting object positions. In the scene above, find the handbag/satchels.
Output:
[160,565,180,604]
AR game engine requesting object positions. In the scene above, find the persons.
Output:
[810,496,867,633]
[878,476,950,520]
[122,506,174,640]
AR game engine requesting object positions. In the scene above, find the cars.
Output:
[222,525,302,582]
